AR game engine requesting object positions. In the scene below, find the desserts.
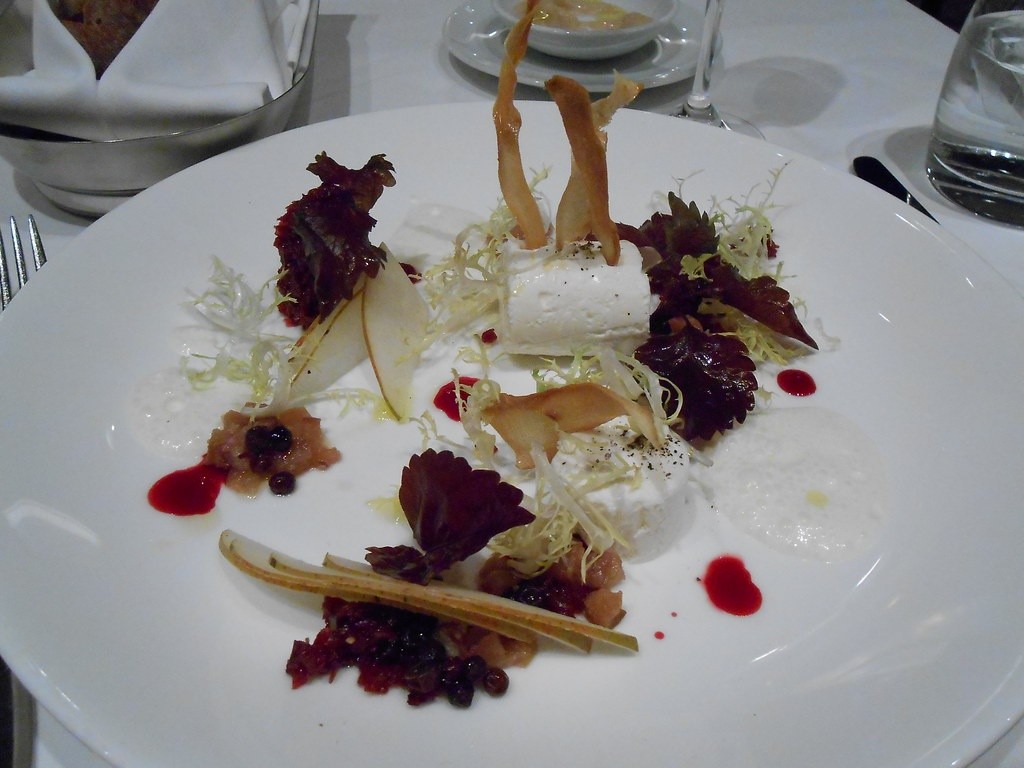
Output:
[147,1,821,711]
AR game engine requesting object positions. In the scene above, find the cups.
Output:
[926,0,1024,226]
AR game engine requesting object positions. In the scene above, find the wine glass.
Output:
[660,1,765,141]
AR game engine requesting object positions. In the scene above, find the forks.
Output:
[0,213,48,768]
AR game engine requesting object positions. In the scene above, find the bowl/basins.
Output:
[492,0,678,60]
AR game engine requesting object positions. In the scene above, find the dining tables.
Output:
[0,0,1024,768]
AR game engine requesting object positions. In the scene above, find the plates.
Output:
[0,100,1022,767]
[442,0,715,93]
